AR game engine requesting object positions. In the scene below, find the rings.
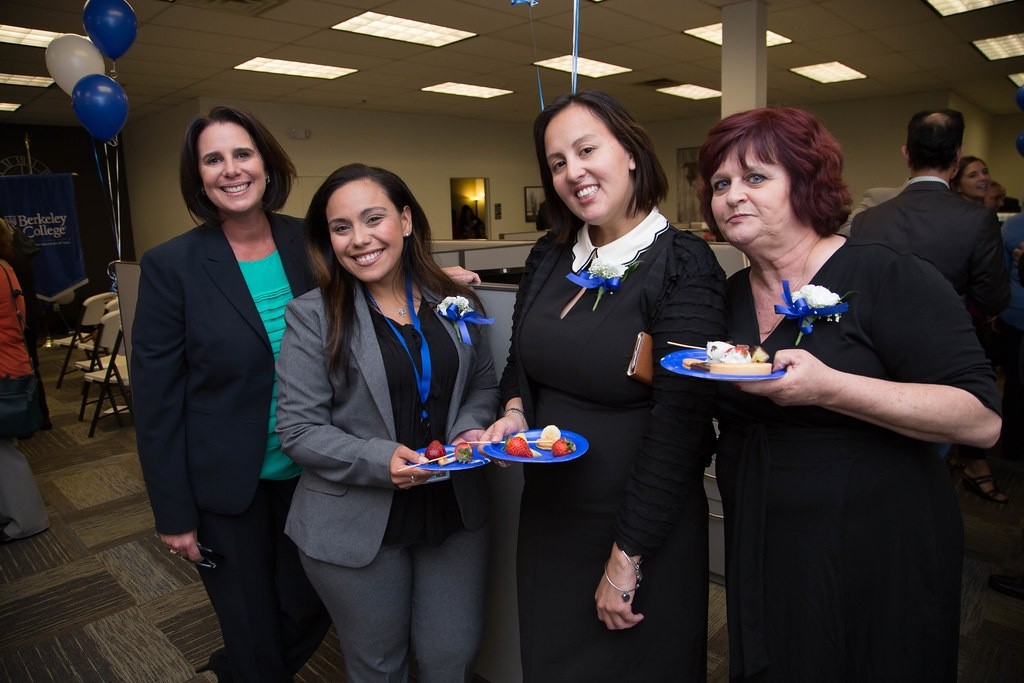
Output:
[410,476,416,484]
[169,550,178,554]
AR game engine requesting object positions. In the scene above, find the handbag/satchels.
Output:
[0,375,44,437]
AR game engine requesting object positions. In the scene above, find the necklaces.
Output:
[753,243,823,335]
[375,298,409,318]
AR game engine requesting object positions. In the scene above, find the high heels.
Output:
[195,652,228,683]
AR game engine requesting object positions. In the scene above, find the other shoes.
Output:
[989,574,1024,598]
[0,527,49,543]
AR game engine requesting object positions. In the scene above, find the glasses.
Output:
[195,548,225,569]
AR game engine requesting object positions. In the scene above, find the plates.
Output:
[482,428,589,462]
[407,445,491,470]
[659,348,786,380]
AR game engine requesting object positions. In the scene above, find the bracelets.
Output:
[502,408,524,416]
[605,561,640,603]
[618,550,641,576]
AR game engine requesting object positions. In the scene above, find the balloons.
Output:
[45,34,105,98]
[1015,84,1024,112]
[1016,131,1024,158]
[71,74,128,142]
[82,0,138,60]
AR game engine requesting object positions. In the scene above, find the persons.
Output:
[479,92,728,683]
[0,218,52,542]
[130,106,352,683]
[849,108,1024,501]
[275,163,502,683]
[456,205,485,238]
[698,108,1003,683]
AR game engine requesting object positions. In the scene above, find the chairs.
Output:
[53,292,134,438]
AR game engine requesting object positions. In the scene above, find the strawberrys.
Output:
[425,440,447,460]
[506,437,534,458]
[454,442,473,463]
[552,438,575,456]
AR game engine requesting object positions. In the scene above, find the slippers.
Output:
[963,474,1009,502]
[946,453,966,469]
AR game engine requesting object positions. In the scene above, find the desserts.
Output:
[681,341,772,376]
